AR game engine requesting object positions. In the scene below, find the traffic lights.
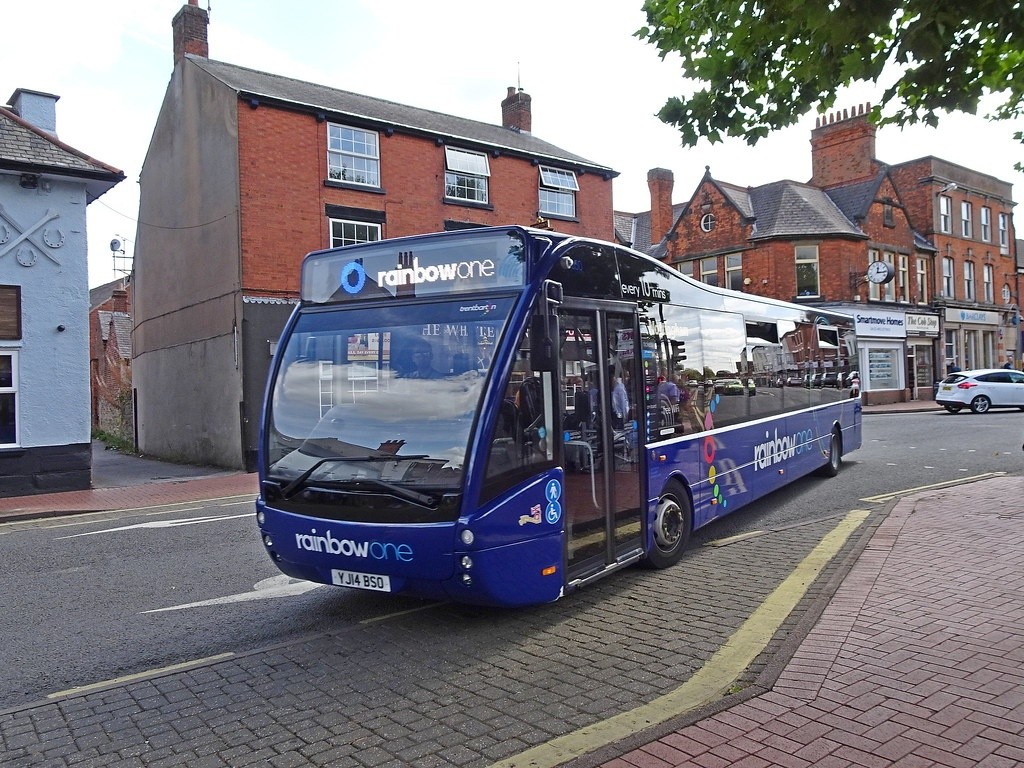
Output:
[670,339,687,363]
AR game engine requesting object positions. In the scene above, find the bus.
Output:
[253,224,864,615]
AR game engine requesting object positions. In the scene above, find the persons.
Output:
[1004,357,1013,369]
[951,362,961,373]
[395,338,455,421]
[611,369,680,437]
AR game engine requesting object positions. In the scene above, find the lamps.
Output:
[20,172,37,189]
[939,182,958,193]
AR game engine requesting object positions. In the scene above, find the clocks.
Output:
[867,261,895,284]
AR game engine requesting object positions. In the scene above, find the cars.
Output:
[775,372,851,390]
[688,379,757,397]
[935,369,1024,414]
